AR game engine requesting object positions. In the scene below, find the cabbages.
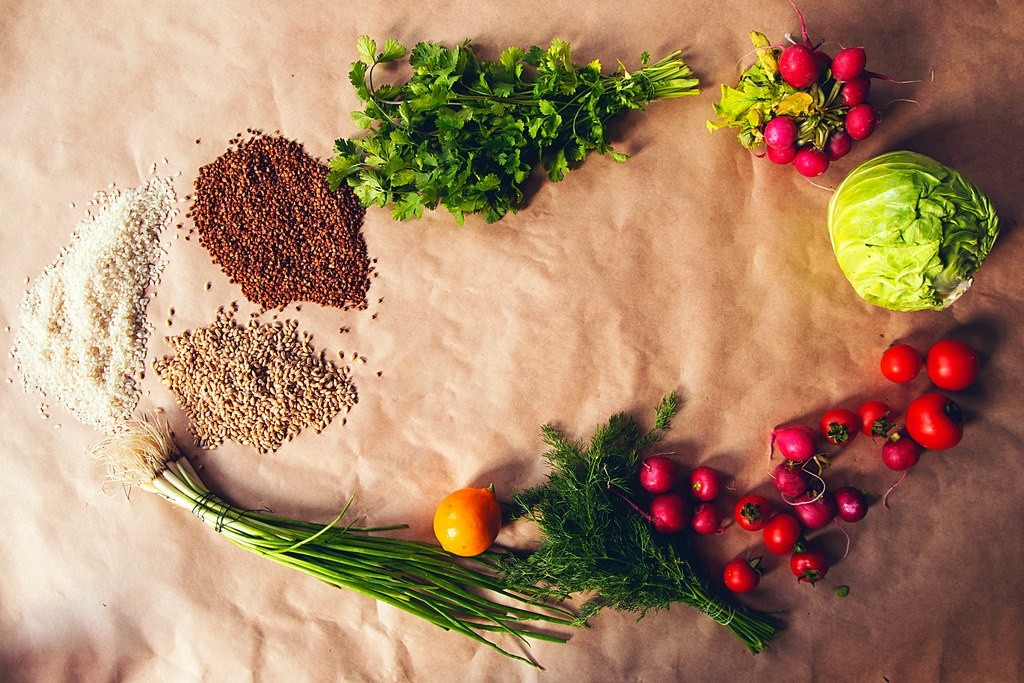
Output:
[826,151,1003,313]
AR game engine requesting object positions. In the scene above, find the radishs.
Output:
[706,0,919,180]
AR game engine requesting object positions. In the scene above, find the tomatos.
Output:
[648,343,978,594]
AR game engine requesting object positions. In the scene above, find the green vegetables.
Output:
[326,33,698,223]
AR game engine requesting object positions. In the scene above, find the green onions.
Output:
[88,414,594,675]
